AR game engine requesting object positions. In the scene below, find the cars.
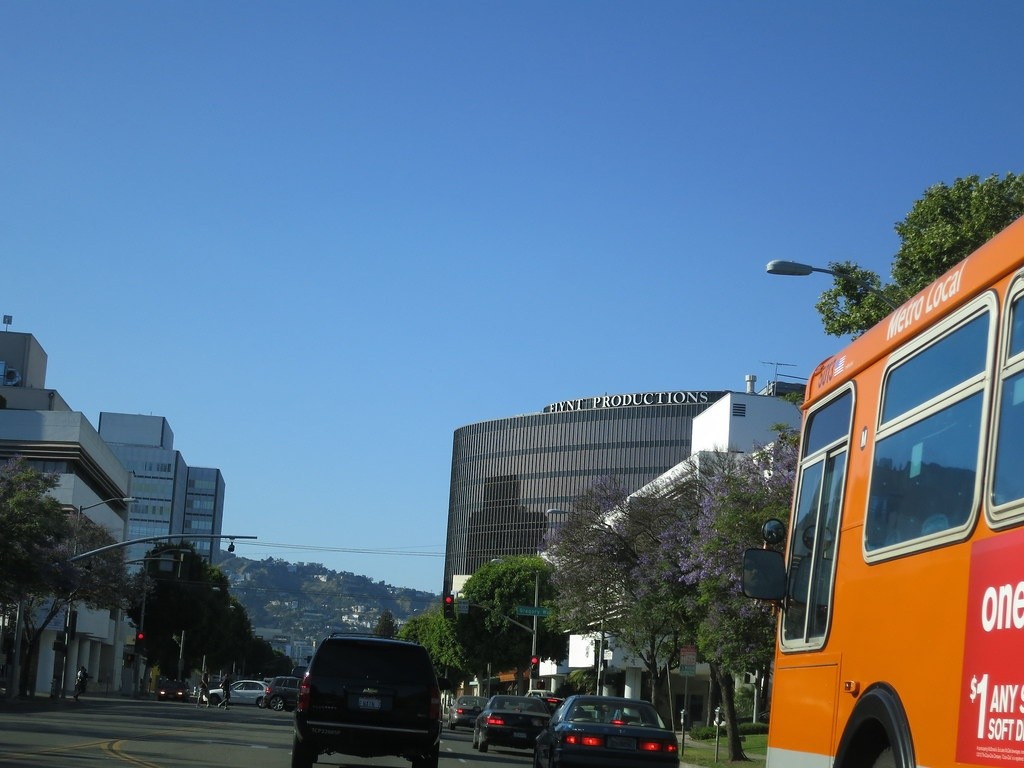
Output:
[532,695,680,768]
[447,694,490,730]
[158,681,190,702]
[473,690,592,753]
[202,679,269,708]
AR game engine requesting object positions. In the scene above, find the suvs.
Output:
[263,665,308,711]
[291,632,451,768]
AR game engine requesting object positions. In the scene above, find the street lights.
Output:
[59,498,140,700]
[546,509,611,720]
[201,605,235,681]
[135,549,191,693]
[413,608,448,714]
[179,587,221,680]
[490,557,540,694]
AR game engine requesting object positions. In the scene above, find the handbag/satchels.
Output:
[199,680,208,688]
[220,683,225,689]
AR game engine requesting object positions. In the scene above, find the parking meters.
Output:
[680,709,688,757]
[714,706,723,764]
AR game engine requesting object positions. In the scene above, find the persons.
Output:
[218,673,230,710]
[743,555,780,598]
[196,668,212,709]
[73,665,94,702]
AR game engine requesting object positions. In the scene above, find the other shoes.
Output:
[196,705,201,708]
[74,695,77,701]
[224,707,230,710]
[206,705,213,709]
[218,703,221,708]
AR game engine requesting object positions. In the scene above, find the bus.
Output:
[741,217,1024,768]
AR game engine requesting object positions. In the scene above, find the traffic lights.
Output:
[442,594,455,619]
[136,630,144,650]
[530,654,540,679]
